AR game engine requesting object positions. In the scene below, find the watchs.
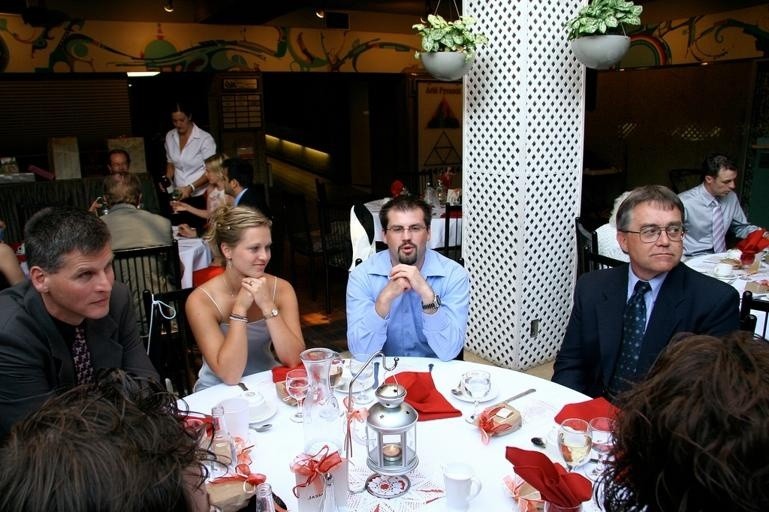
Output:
[263,308,280,320]
[421,293,442,312]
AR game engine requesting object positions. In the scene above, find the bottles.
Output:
[254,482,274,512]
[158,170,175,198]
[299,346,341,456]
[171,192,180,199]
[318,474,341,512]
[425,182,433,204]
[209,405,237,474]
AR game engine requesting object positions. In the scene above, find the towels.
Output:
[553,394,630,434]
[382,370,463,422]
[504,442,594,507]
[178,412,221,436]
[271,361,310,383]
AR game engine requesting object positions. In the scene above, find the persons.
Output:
[184,204,306,393]
[591,328,769,512]
[676,153,769,263]
[91,150,183,336]
[0,204,163,441]
[0,366,232,511]
[165,103,216,237]
[0,218,27,286]
[345,195,470,363]
[551,183,739,403]
[172,155,262,220]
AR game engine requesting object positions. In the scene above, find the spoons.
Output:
[248,425,272,434]
[531,437,547,445]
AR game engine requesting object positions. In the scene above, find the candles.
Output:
[382,443,403,462]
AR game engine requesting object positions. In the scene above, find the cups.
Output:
[236,390,267,418]
[442,468,482,512]
[713,263,733,276]
[743,253,763,275]
[556,417,594,470]
[329,351,344,387]
[217,397,250,445]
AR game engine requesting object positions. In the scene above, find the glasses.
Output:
[622,221,683,243]
[384,224,427,232]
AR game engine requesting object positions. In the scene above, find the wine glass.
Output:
[588,417,618,481]
[463,369,490,426]
[171,200,179,214]
[350,354,375,405]
[285,369,311,424]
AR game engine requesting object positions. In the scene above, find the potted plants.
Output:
[565,1,644,72]
[409,1,487,82]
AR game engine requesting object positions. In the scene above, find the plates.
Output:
[717,273,739,280]
[448,382,497,404]
[335,358,377,394]
[250,406,278,424]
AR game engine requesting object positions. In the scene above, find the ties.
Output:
[71,325,96,383]
[607,279,652,402]
[711,200,729,253]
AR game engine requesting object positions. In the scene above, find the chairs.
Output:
[576,164,769,338]
[143,285,205,399]
[263,169,462,314]
[111,238,193,392]
[2,172,207,261]
[354,257,466,360]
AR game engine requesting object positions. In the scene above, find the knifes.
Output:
[373,362,380,391]
[502,388,537,405]
